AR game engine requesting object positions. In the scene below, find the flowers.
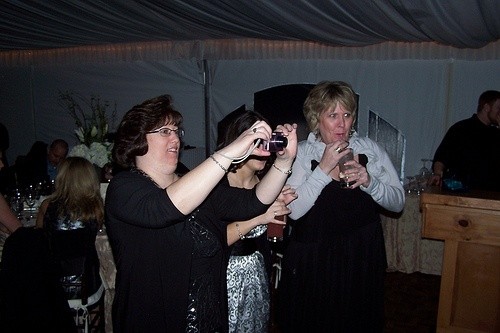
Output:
[67,96,116,167]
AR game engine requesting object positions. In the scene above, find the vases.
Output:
[94,164,105,183]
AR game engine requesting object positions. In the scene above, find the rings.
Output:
[357,173,361,177]
[253,128,256,133]
[336,147,342,153]
[274,212,277,216]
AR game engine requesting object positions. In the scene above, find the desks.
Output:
[380,189,443,276]
[422,179,500,333]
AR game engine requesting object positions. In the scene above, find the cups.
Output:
[267,216,284,242]
[10,178,55,220]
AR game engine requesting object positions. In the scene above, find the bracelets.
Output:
[236,224,245,239]
[431,172,441,177]
[273,163,292,174]
[210,156,228,173]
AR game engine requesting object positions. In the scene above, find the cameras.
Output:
[264,131,288,152]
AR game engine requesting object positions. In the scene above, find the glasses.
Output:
[146,128,185,138]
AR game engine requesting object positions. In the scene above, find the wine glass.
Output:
[403,159,440,197]
[338,148,354,188]
[11,196,24,220]
[26,194,35,219]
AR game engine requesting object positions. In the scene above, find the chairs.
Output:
[0,226,95,333]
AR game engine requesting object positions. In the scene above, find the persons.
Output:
[104,93,297,333]
[431,90,500,187]
[32,139,67,192]
[275,80,405,333]
[0,151,22,232]
[36,155,104,313]
[225,111,298,333]
